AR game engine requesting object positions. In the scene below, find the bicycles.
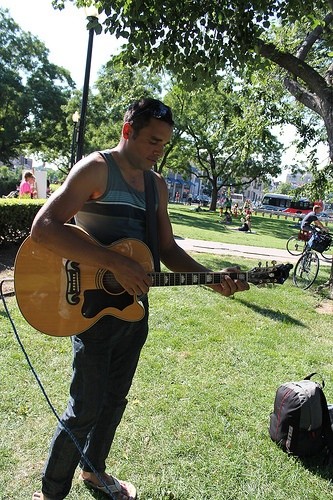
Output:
[286,227,333,260]
[293,226,333,290]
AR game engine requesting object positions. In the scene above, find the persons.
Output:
[175,192,253,232]
[2,173,50,199]
[301,205,330,234]
[30,98,250,500]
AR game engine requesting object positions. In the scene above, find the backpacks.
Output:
[269,372,333,458]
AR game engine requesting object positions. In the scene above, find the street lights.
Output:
[76,5,99,163]
[71,111,80,168]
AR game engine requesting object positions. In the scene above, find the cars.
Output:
[192,195,210,203]
[256,206,333,222]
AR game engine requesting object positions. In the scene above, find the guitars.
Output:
[13,223,294,338]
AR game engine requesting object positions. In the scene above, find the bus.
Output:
[258,193,325,215]
[219,193,243,209]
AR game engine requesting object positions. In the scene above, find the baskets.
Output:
[308,235,330,253]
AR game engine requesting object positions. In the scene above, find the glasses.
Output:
[129,105,169,123]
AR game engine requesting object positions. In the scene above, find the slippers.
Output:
[32,490,47,500]
[83,474,136,500]
